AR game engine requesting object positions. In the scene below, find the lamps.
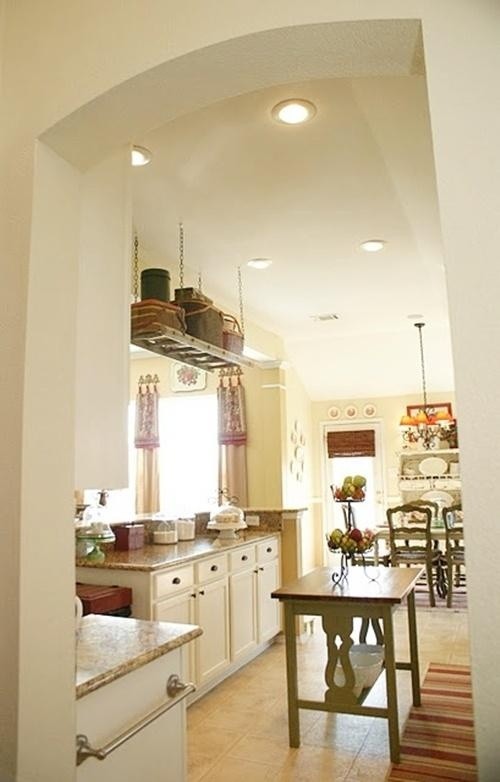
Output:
[400,324,458,450]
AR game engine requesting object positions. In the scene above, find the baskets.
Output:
[223,314,243,354]
[170,301,223,349]
[131,299,187,331]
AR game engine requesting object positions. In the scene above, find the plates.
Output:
[374,523,397,528]
[419,492,459,527]
[287,420,310,482]
[328,402,377,420]
[403,456,448,475]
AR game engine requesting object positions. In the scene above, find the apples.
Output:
[335,476,366,501]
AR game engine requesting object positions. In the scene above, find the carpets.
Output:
[383,662,477,782]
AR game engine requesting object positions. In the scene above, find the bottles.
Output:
[149,508,198,546]
[140,267,171,302]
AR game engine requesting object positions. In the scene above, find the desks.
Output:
[272,564,420,763]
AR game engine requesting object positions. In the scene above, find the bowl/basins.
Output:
[333,642,386,691]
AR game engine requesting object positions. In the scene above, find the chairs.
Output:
[375,499,466,608]
